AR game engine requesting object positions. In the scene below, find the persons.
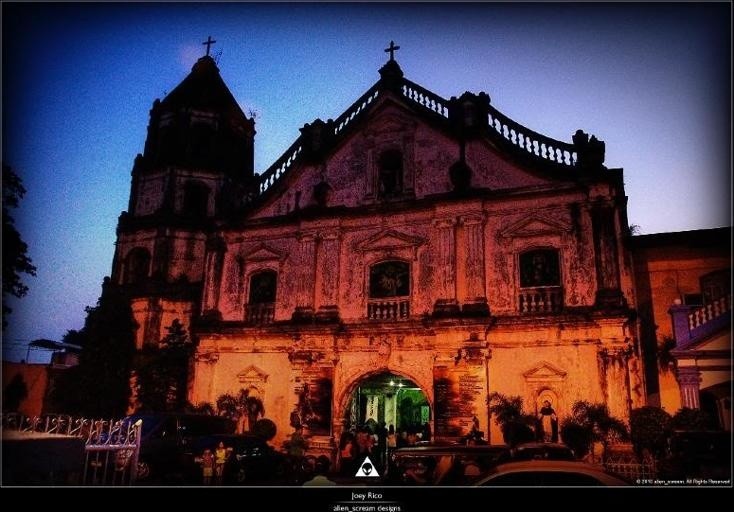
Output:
[539,399,557,440]
[337,421,481,487]
[300,454,339,487]
[281,423,305,482]
[214,439,231,482]
[193,447,215,485]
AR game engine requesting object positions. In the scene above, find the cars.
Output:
[508,441,577,463]
[467,461,632,487]
